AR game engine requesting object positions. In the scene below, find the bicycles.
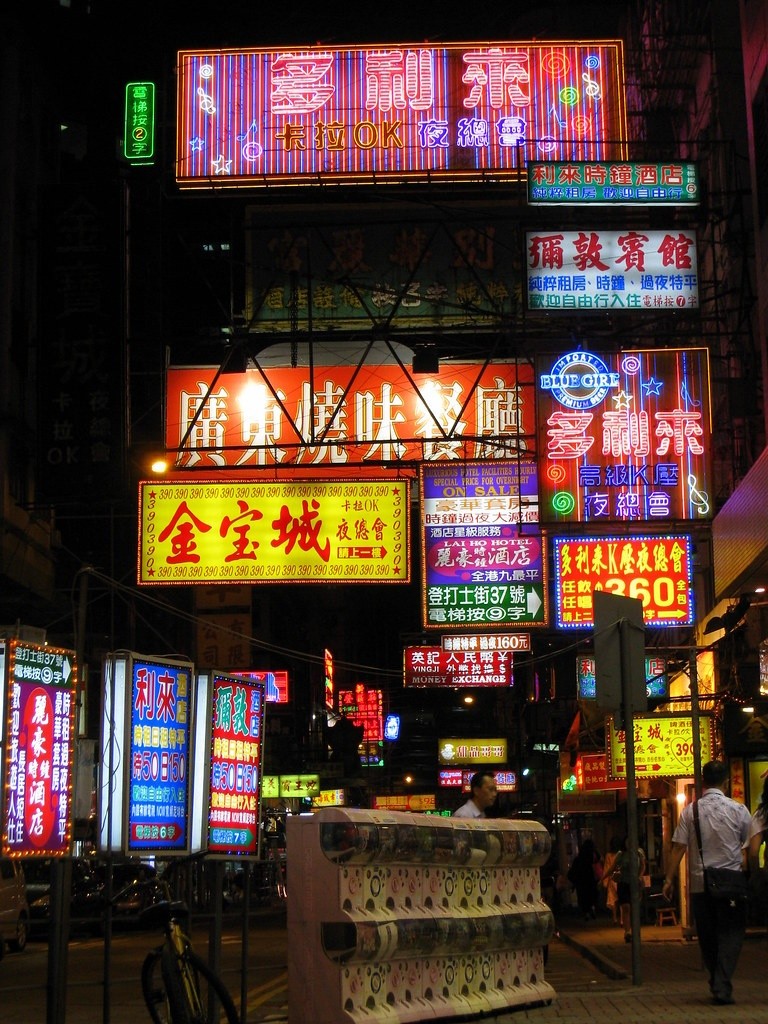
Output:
[104,844,239,1024]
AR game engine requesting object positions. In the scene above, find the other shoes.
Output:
[712,997,735,1006]
[625,932,631,945]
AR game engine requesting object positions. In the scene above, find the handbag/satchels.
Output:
[703,866,750,904]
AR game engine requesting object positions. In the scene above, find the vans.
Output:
[1,851,34,950]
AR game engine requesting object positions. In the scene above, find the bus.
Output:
[89,861,159,925]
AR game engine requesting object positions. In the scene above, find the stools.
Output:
[656,907,677,927]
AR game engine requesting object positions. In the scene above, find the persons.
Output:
[663,760,768,1005]
[450,770,497,818]
[541,822,646,943]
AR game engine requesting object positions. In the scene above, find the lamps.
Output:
[754,701,768,718]
[702,590,761,638]
